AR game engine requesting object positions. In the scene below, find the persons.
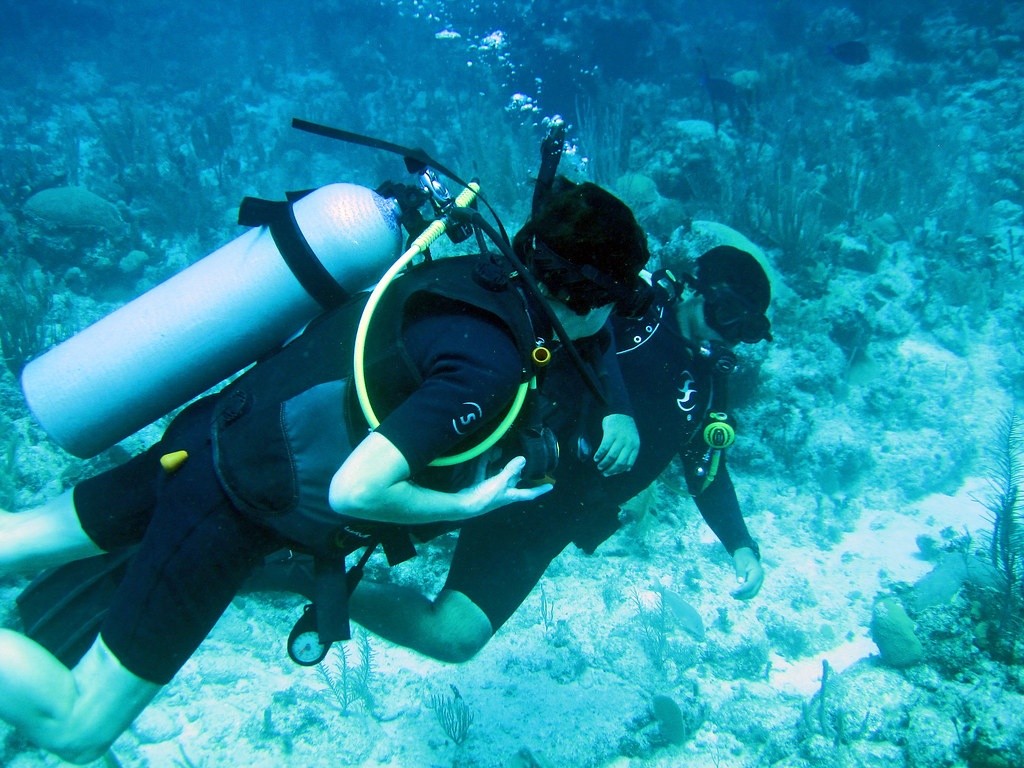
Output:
[0,174,651,766]
[237,245,773,667]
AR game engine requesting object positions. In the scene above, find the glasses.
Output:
[535,236,657,320]
[706,285,772,343]
